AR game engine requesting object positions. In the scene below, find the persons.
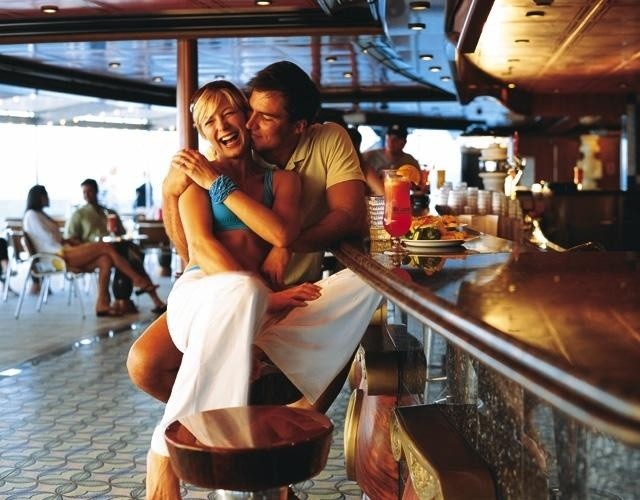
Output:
[23,184,160,316]
[30,268,54,294]
[146,79,384,500]
[0,238,17,291]
[348,128,384,240]
[65,178,168,314]
[322,107,350,131]
[123,61,372,405]
[358,123,422,173]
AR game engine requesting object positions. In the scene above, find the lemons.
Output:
[397,165,422,187]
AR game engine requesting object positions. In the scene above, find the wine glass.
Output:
[380,169,412,255]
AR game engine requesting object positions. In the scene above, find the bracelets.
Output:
[209,174,242,202]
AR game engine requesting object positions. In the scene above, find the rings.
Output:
[177,163,184,169]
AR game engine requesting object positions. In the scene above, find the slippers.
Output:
[97,283,166,318]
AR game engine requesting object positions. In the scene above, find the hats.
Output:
[387,124,407,138]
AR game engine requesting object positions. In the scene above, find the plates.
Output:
[437,181,524,217]
[403,247,468,253]
[403,237,466,248]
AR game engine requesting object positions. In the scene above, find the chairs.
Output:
[2,222,86,322]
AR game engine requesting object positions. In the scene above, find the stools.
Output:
[163,400,336,500]
